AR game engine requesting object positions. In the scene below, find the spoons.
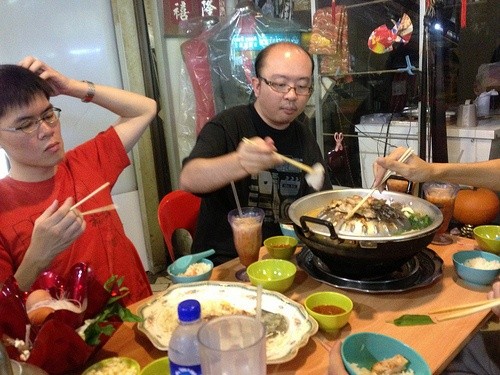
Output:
[169,248,216,275]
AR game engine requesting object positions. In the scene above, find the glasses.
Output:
[0,106,62,134]
[257,76,313,95]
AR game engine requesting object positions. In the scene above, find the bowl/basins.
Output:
[246,258,297,294]
[140,357,170,375]
[452,250,500,288]
[166,259,214,284]
[304,291,353,332]
[340,332,431,375]
[81,356,140,375]
[472,225,500,254]
[263,219,301,260]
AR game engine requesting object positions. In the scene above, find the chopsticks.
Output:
[428,298,500,322]
[70,181,119,215]
[345,146,414,220]
[241,136,314,174]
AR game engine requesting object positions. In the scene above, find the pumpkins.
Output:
[452,184,499,225]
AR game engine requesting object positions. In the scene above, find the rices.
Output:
[463,257,500,270]
[349,362,414,375]
[85,357,137,375]
[176,263,212,277]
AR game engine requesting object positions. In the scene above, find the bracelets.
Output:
[80,80,95,103]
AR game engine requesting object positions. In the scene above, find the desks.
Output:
[77,235,500,375]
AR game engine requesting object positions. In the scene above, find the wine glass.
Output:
[227,206,265,282]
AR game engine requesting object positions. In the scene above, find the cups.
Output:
[196,315,267,375]
[423,182,457,245]
[385,175,417,196]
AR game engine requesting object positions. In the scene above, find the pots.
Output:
[288,187,444,276]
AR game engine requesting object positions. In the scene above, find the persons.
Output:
[370,147,500,318]
[0,56,157,349]
[178,41,333,270]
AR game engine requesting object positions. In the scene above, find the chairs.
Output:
[158,190,203,261]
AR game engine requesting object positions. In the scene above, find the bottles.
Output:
[167,299,204,375]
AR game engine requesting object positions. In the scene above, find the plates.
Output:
[136,280,319,364]
